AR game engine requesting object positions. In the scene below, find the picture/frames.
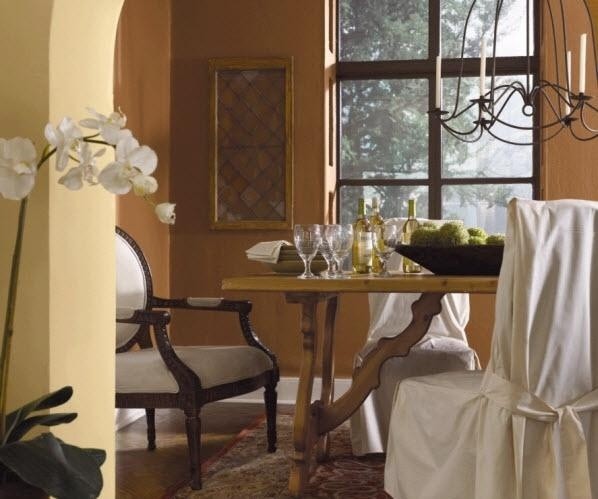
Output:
[207,54,294,235]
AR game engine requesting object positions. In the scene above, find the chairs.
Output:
[350,218,480,457]
[385,194,598,499]
[114,232,278,489]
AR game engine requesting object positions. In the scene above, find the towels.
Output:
[241,239,293,265]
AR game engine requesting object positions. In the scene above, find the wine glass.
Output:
[372,223,397,278]
[293,224,352,280]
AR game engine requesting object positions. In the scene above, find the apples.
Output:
[467,228,504,246]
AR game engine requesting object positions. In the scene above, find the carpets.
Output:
[169,411,384,498]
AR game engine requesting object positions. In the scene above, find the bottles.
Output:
[401,197,421,273]
[353,195,384,273]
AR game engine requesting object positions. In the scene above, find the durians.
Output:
[409,222,467,248]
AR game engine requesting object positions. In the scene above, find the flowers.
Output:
[0,104,172,421]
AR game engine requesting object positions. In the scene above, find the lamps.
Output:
[426,0,598,145]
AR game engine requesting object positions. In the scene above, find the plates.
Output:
[268,243,341,274]
[397,245,503,275]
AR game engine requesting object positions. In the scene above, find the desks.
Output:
[224,278,501,499]
